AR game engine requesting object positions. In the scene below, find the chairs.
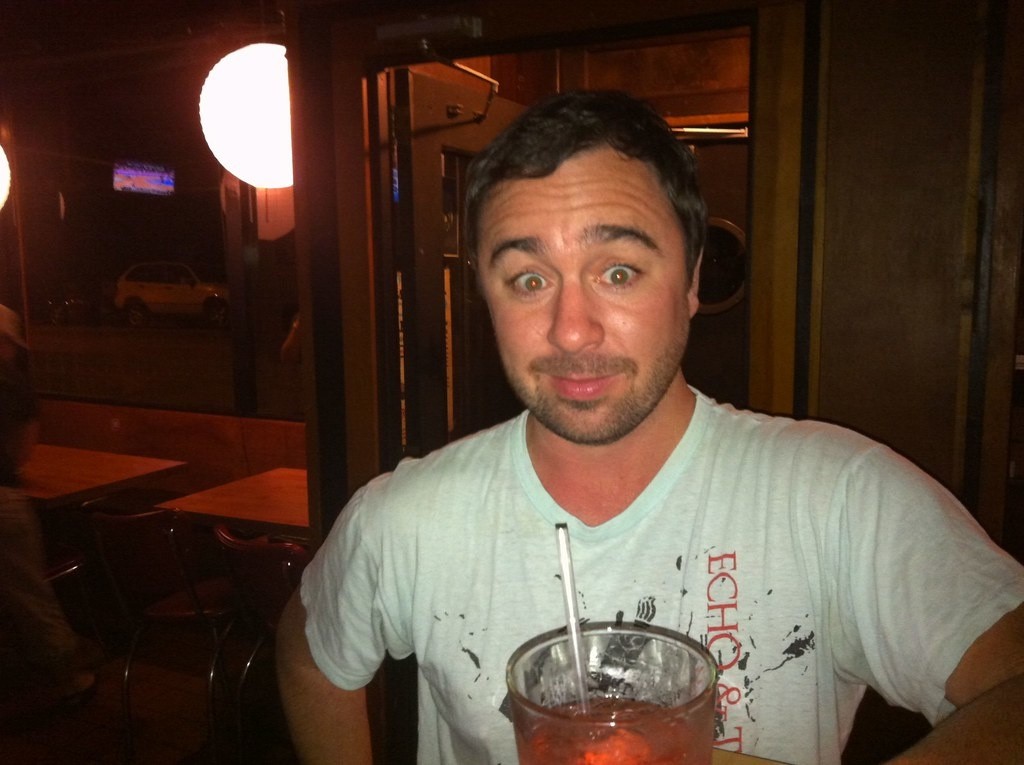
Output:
[89,511,311,765]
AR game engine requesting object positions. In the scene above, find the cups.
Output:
[504,619,719,765]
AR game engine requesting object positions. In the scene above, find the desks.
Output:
[19,444,312,548]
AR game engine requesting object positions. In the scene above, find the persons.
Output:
[0,303,101,705]
[280,89,1024,764]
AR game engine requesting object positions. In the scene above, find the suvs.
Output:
[115,259,229,329]
